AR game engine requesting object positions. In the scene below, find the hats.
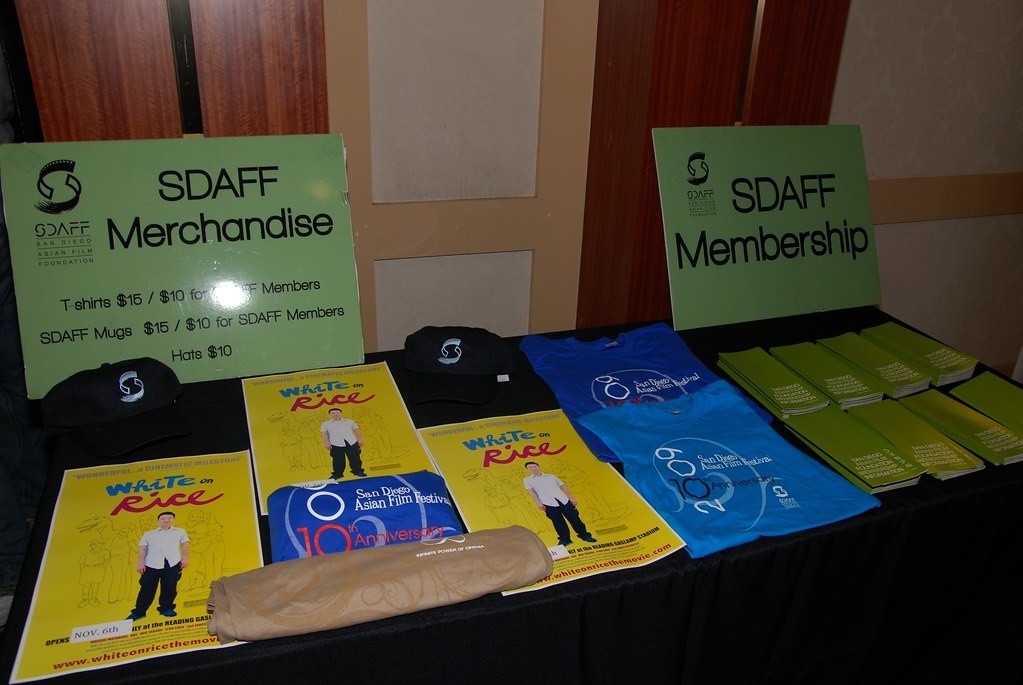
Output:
[402,325,513,404]
[40,357,190,458]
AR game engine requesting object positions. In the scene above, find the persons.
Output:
[321,408,367,480]
[523,462,597,547]
[126,512,191,621]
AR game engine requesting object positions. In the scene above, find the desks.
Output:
[0,307,1023,684]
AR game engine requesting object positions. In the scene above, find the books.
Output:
[8,449,265,684]
[241,360,436,515]
[716,322,1023,495]
[949,370,1023,433]
[416,405,688,597]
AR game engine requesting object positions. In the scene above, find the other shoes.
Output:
[125,614,146,622]
[583,536,596,542]
[160,609,176,616]
[557,540,573,547]
[329,475,343,480]
[356,472,366,476]
